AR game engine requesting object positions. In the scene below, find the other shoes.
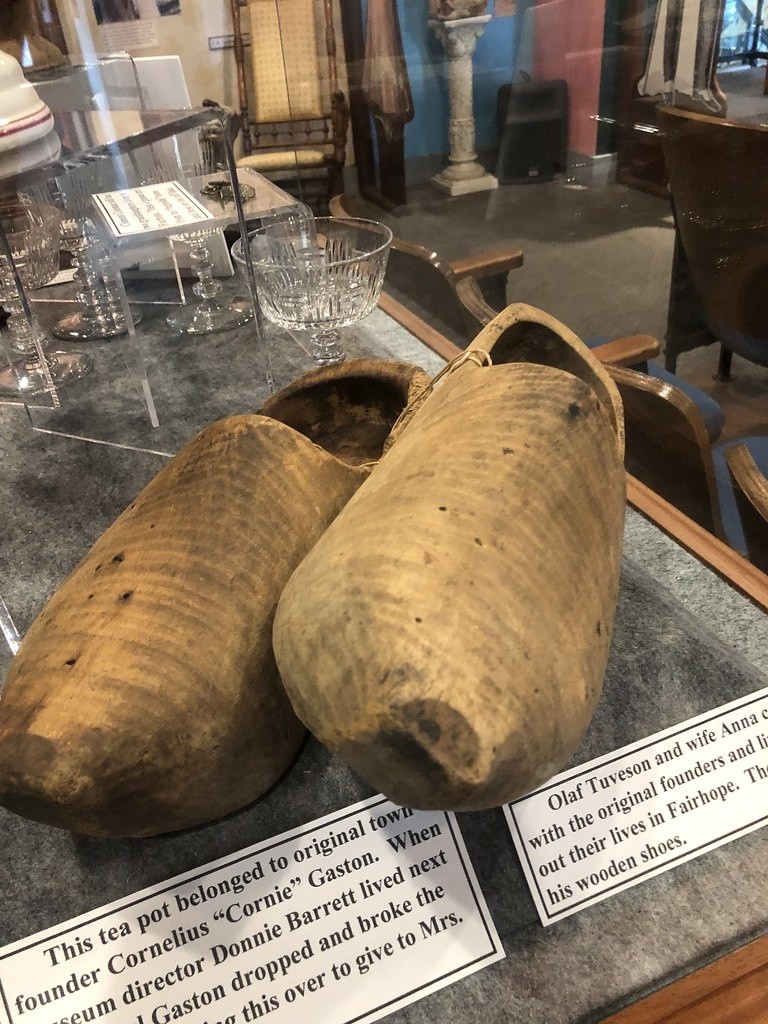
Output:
[0,304,624,840]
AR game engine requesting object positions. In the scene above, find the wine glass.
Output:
[0,205,93,398]
[129,141,255,335]
[231,216,394,365]
[17,176,144,340]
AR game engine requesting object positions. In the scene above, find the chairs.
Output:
[218,0,768,577]
